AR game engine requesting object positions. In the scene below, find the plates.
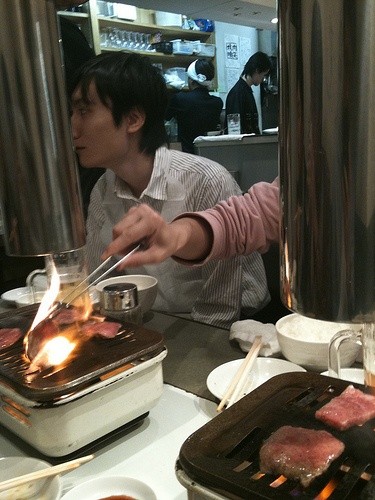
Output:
[320,368,365,385]
[1,286,33,304]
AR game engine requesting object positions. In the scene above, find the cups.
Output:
[327,323,375,388]
[26,248,93,313]
[227,114,240,135]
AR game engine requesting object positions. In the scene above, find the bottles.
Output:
[100,282,143,325]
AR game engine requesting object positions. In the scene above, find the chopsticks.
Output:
[0,454,94,492]
[215,336,263,412]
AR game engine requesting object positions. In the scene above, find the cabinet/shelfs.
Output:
[57,0,219,90]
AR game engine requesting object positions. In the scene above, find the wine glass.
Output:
[99,26,152,52]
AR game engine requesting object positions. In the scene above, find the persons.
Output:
[68,50,271,330]
[166,59,223,154]
[101,175,281,270]
[226,51,272,134]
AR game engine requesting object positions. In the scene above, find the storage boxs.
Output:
[195,43,216,57]
[169,39,193,55]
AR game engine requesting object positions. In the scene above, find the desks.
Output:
[194,133,278,191]
[0,310,363,500]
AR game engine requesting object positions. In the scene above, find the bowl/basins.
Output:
[275,313,361,372]
[95,274,158,314]
[60,475,157,500]
[0,456,62,500]
[15,293,33,309]
[205,357,307,406]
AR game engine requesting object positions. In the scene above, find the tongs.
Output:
[29,242,144,336]
